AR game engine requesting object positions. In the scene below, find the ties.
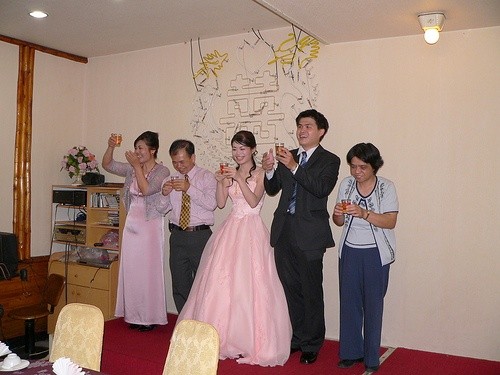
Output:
[179,175,191,230]
[287,152,307,216]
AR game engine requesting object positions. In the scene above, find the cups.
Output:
[220,163,228,174]
[171,176,179,184]
[275,143,284,157]
[342,199,351,215]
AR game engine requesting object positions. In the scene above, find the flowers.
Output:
[59,144,100,179]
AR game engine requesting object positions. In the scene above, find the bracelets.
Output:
[364,211,370,220]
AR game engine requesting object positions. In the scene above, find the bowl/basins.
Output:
[0,341,12,356]
[3,353,22,369]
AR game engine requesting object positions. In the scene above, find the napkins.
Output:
[51,357,86,375]
[0,341,12,357]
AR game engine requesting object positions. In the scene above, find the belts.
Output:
[173,224,209,232]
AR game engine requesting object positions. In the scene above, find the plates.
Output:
[0,359,30,372]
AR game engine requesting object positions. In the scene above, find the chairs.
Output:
[162,320,220,375]
[49,302,106,373]
[8,273,67,360]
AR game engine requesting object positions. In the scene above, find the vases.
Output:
[71,170,84,185]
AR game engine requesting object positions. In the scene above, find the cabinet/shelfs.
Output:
[48,183,127,336]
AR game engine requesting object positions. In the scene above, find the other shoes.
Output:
[129,324,140,329]
[338,357,364,367]
[138,325,158,331]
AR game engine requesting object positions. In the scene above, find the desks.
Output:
[0,358,105,375]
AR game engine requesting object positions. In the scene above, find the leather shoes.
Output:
[300,352,318,364]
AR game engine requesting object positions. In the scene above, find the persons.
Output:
[176,130,292,367]
[332,142,399,372]
[262,109,341,364]
[156,140,217,313]
[102,131,170,332]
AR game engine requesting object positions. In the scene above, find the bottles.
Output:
[111,133,122,147]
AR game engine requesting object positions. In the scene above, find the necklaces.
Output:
[146,163,158,180]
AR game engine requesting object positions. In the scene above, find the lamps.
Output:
[417,11,447,45]
